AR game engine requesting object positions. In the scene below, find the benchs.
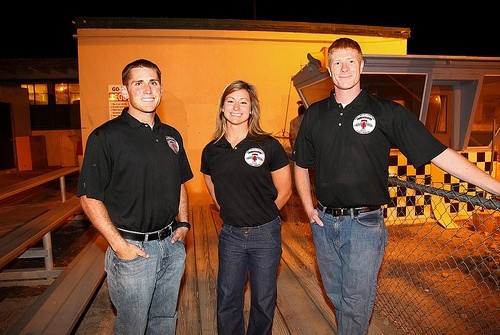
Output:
[0,168,79,200]
[0,194,82,272]
[3,232,109,335]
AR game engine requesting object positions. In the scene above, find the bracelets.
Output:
[176,222,191,231]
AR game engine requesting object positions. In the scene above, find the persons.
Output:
[289,38,500,335]
[76,59,194,335]
[200,80,293,335]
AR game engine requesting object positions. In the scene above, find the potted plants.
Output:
[473,119,500,233]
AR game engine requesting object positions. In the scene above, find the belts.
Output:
[316,200,381,217]
[117,219,178,242]
